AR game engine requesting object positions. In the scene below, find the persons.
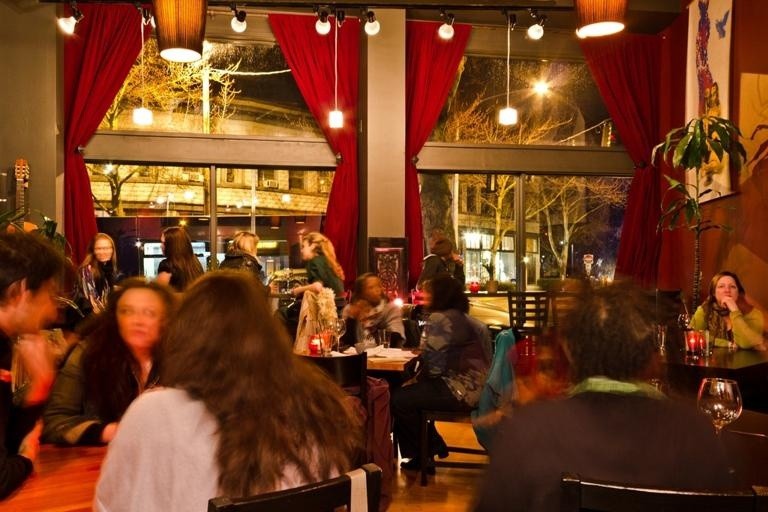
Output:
[686,268,764,357]
[78,233,124,314]
[0,233,80,506]
[392,275,493,477]
[217,232,273,306]
[577,253,598,277]
[157,227,205,292]
[416,237,465,290]
[39,277,179,448]
[289,222,313,269]
[463,280,741,512]
[342,272,405,350]
[93,270,368,512]
[291,232,350,315]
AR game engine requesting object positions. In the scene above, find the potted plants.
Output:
[483,262,498,294]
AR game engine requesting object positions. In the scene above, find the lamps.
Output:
[438,8,455,40]
[230,2,247,32]
[499,11,518,126]
[57,0,84,34]
[132,1,156,125]
[528,9,549,40]
[574,0,628,38]
[154,0,208,63]
[315,6,331,35]
[364,11,380,35]
[328,7,344,128]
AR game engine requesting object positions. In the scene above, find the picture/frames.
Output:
[684,0,740,206]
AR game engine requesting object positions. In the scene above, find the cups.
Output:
[378,327,391,353]
[648,380,664,391]
[652,322,668,353]
[699,330,710,359]
[685,330,697,358]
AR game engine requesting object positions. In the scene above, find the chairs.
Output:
[656,287,688,321]
[550,290,585,328]
[560,477,768,512]
[208,463,382,511]
[335,296,348,317]
[508,290,549,337]
[296,352,367,412]
[420,324,510,487]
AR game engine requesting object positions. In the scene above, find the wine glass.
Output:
[330,319,347,354]
[696,377,742,473]
[678,315,691,353]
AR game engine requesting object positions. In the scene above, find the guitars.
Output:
[6,158,41,231]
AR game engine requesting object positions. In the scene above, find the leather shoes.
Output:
[424,436,449,459]
[401,459,436,475]
